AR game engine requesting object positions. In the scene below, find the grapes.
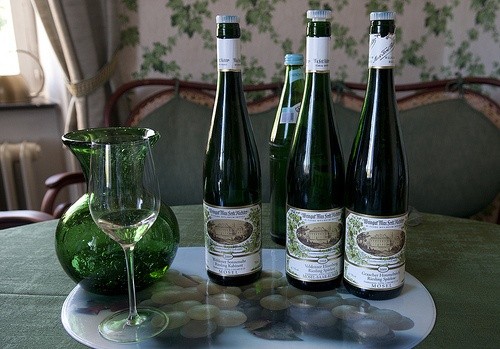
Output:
[139,269,414,345]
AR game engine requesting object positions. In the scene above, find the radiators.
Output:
[0,140,42,211]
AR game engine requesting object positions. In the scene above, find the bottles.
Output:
[268,53,306,246]
[342,9,409,300]
[203,14,263,287]
[53,126,181,298]
[283,9,346,293]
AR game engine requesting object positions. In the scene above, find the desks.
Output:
[0,204,500,349]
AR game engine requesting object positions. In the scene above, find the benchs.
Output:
[40,76,500,223]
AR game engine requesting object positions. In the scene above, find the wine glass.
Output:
[86,135,170,343]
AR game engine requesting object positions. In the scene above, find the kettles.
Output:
[0,49,46,105]
